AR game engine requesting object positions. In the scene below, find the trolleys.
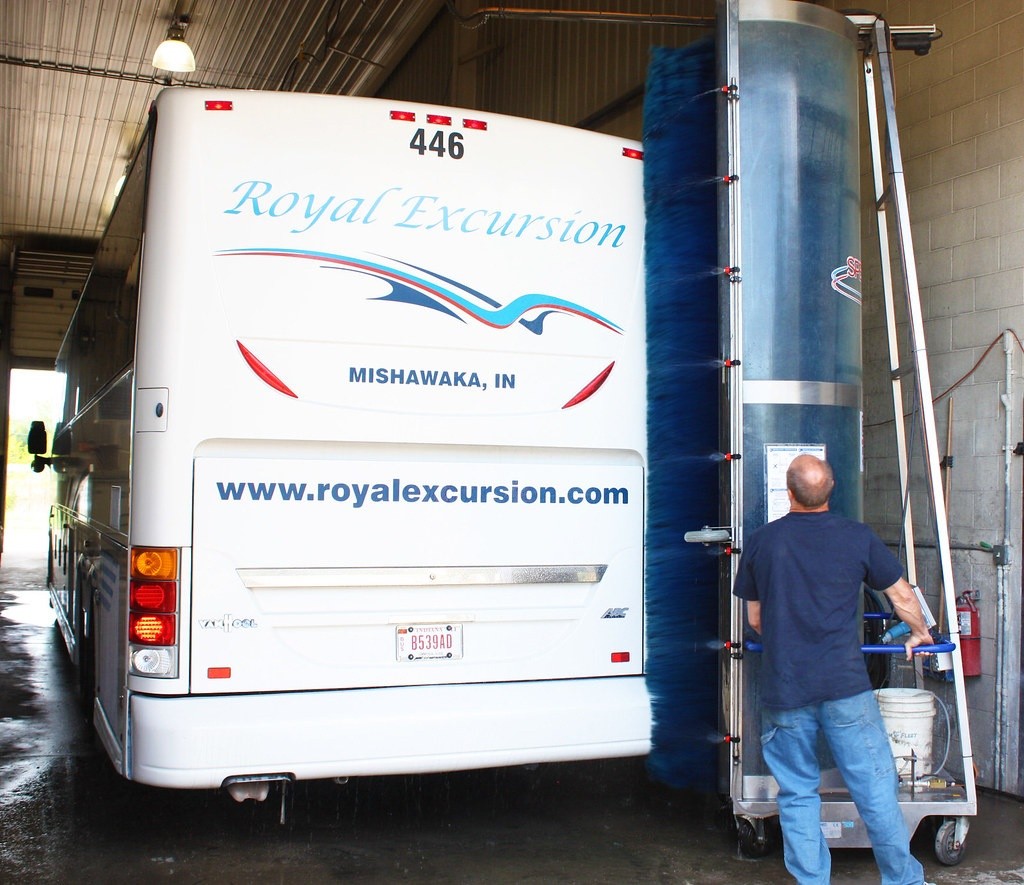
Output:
[719,3,985,861]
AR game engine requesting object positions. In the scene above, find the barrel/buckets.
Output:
[872,687,936,781]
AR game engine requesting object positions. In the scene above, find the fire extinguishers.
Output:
[955,589,982,678]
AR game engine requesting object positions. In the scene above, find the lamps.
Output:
[151,25,195,73]
[114,174,126,197]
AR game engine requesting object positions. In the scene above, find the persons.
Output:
[731,453,936,885]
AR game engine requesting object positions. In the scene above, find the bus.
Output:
[26,79,650,811]
